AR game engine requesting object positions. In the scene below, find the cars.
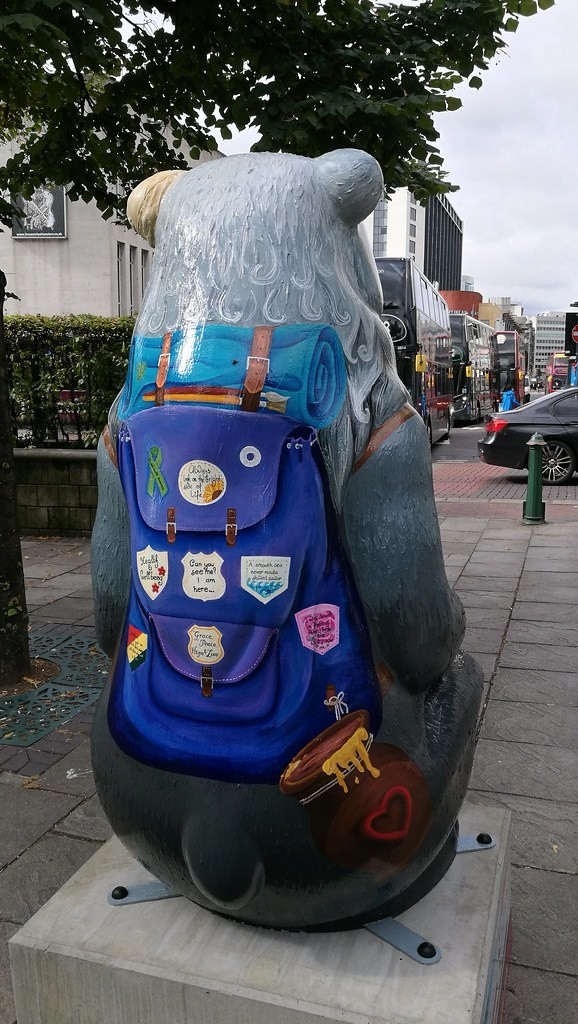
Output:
[477,387,578,484]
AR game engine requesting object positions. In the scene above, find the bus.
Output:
[449,314,495,424]
[546,354,570,394]
[492,330,526,412]
[374,254,454,445]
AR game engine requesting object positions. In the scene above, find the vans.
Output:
[523,376,531,403]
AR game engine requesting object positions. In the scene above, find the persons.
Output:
[500,384,521,411]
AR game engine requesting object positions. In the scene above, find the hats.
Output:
[507,384,516,390]
